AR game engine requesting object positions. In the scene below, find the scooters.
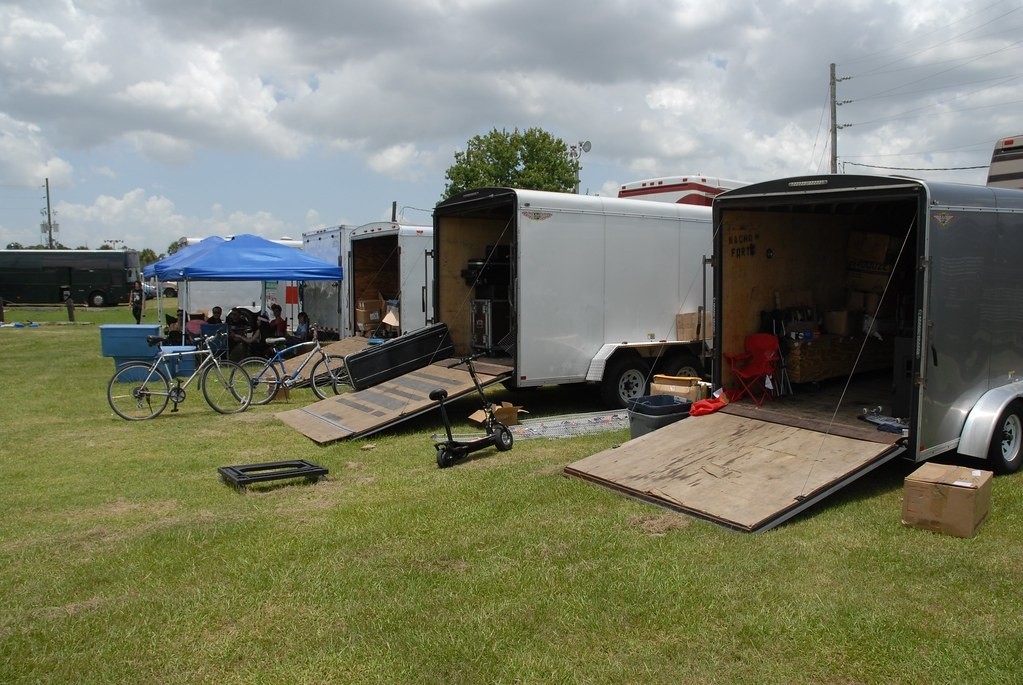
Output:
[430,351,514,469]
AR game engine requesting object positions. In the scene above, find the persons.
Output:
[176,304,309,368]
[129,281,146,324]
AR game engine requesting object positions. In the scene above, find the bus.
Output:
[1,250,141,307]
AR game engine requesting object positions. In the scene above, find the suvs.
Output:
[141,274,178,298]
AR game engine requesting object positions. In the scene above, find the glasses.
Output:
[213,311,220,314]
[176,313,183,316]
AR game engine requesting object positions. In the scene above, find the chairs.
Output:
[165,311,311,368]
[723,334,779,406]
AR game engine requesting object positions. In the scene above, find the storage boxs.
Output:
[628,410,690,437]
[357,299,381,309]
[900,462,994,539]
[654,375,702,386]
[161,346,195,376]
[356,308,380,323]
[470,299,510,356]
[626,394,693,415]
[823,231,900,336]
[98,323,159,358]
[468,402,529,426]
[113,358,162,383]
[650,383,700,402]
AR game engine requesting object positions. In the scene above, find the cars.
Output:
[141,282,160,300]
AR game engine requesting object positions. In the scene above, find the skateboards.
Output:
[857,405,910,428]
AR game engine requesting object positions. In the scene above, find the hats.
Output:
[258,314,270,322]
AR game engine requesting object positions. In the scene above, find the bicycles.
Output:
[230,320,353,404]
[105,328,254,422]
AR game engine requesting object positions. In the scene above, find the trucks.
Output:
[270,183,714,445]
[564,174,1022,535]
[256,221,435,394]
[177,237,304,320]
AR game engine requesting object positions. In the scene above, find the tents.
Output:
[159,234,344,346]
[143,236,227,336]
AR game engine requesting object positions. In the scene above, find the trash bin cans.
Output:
[627,395,693,440]
